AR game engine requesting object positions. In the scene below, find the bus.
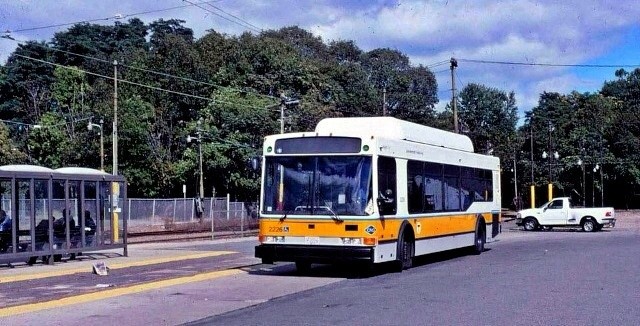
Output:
[254,113,502,274]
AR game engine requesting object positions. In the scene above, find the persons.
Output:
[26,216,55,266]
[0,210,12,254]
[352,177,372,215]
[68,210,96,260]
[195,193,204,223]
[54,209,75,262]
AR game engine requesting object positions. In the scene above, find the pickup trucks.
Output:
[515,196,617,231]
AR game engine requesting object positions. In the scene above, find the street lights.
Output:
[593,162,604,207]
[577,158,586,205]
[186,130,204,199]
[541,119,560,202]
[86,118,104,170]
